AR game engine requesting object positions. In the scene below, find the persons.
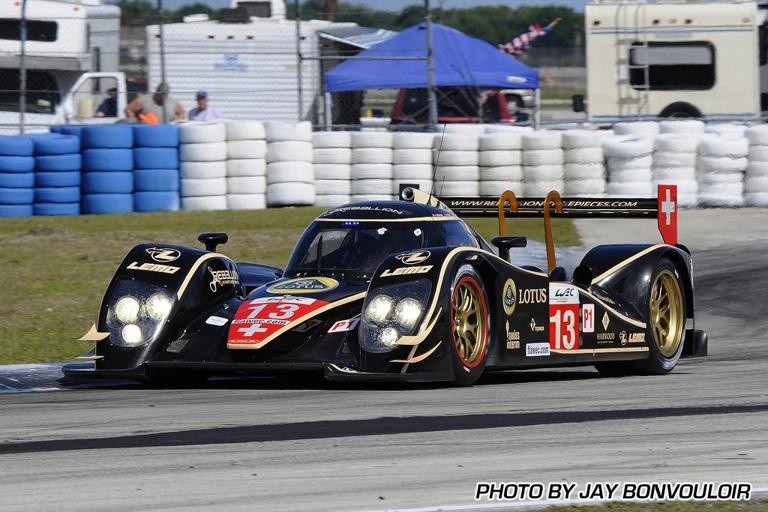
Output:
[481,88,516,124]
[124,84,185,125]
[92,85,117,117]
[188,90,220,122]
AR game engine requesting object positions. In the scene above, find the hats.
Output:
[196,92,208,97]
[138,113,158,125]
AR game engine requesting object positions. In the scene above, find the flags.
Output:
[495,17,566,59]
